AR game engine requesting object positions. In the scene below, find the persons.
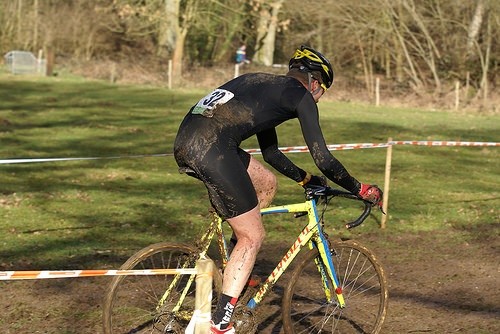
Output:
[175,44,385,334]
[235,44,250,64]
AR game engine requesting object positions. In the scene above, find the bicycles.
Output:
[102,165,389,334]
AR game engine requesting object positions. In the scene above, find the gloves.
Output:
[303,175,326,196]
[358,181,383,207]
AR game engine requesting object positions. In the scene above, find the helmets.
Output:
[289,45,333,94]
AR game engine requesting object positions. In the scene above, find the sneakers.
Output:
[208,320,236,334]
[245,274,261,289]
